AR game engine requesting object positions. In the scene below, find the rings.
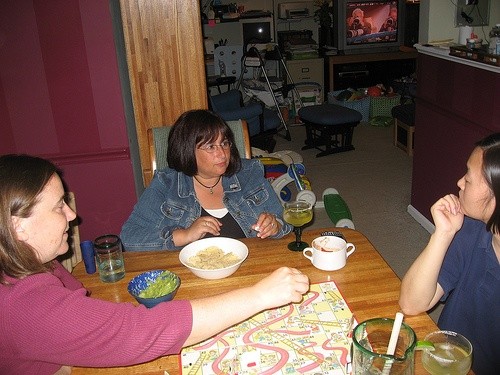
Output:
[272,223,274,225]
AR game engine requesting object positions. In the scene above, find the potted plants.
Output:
[313,0,335,29]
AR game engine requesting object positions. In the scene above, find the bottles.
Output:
[488,24,500,55]
[468,37,475,50]
[93,234,127,283]
[80,240,97,274]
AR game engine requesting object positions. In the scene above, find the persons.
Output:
[398,132,500,375]
[379,7,397,40]
[120,109,294,252]
[0,153,309,375]
[347,9,371,36]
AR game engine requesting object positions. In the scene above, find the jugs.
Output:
[349,317,417,375]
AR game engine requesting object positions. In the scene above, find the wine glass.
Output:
[282,199,313,252]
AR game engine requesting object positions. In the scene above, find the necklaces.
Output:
[194,175,221,194]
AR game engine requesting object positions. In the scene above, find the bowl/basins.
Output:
[466,39,483,48]
[178,237,248,280]
[127,269,180,308]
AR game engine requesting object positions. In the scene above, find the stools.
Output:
[298,103,362,158]
[392,102,415,152]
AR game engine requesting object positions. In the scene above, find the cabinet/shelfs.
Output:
[285,58,325,98]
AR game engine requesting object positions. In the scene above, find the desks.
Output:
[67,226,476,375]
[326,51,417,92]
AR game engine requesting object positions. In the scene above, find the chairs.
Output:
[236,44,306,142]
[143,119,253,187]
[208,76,280,153]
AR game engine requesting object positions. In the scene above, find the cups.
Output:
[302,236,355,271]
[420,330,473,375]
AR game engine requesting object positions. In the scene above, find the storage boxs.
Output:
[327,79,416,123]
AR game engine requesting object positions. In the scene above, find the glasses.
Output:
[197,141,233,153]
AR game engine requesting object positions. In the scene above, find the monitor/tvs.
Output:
[238,17,274,52]
[334,0,404,54]
